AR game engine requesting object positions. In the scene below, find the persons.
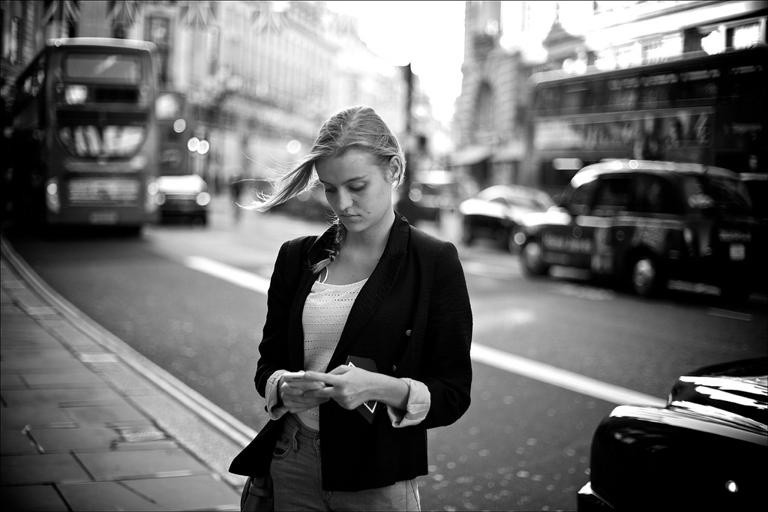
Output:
[227,106,473,511]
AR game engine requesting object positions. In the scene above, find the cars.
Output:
[457,183,561,256]
[575,357,767,512]
[508,156,762,300]
[150,171,212,228]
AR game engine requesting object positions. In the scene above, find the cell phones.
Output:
[280,372,326,396]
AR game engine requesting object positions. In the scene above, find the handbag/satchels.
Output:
[241,477,274,512]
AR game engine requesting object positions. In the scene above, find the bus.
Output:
[0,36,163,240]
